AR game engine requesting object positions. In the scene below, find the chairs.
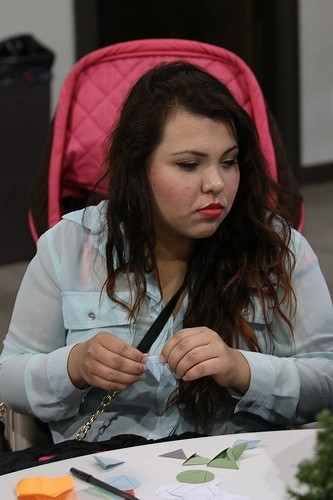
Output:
[29,40,304,242]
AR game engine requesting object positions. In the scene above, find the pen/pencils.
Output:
[70,466,140,500]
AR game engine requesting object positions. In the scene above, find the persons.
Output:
[0,58,333,445]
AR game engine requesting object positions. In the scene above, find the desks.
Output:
[0,425,333,500]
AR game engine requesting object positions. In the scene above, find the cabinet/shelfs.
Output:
[0,32,55,265]
